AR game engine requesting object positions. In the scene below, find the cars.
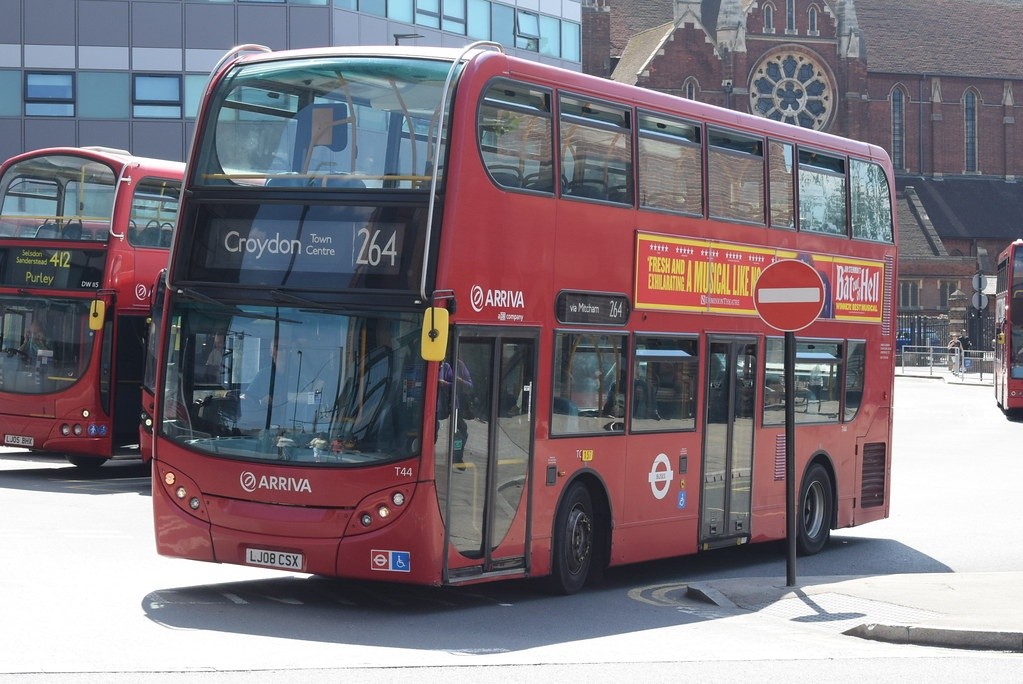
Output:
[896,328,944,360]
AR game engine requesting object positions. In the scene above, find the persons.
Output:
[947,328,973,372]
[437,360,473,470]
[202,329,231,383]
[16,320,56,363]
[242,336,304,407]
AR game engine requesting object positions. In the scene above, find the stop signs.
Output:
[753,261,824,330]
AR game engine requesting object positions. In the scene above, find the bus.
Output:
[995,240,1023,414]
[152,40,898,596]
[0,145,267,470]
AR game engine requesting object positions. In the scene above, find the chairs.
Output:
[263,165,366,190]
[529,379,691,430]
[424,158,839,231]
[29,218,175,251]
